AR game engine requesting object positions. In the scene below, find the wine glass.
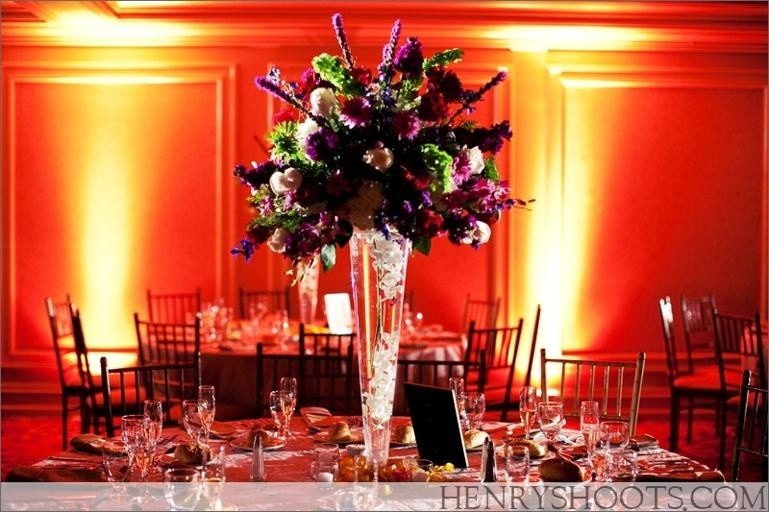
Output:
[98,384,228,511]
[268,390,296,442]
[448,375,466,419]
[445,389,640,512]
[278,376,296,436]
[193,294,425,351]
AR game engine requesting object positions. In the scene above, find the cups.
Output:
[308,410,436,511]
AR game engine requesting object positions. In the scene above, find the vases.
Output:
[347,230,410,471]
[296,259,319,327]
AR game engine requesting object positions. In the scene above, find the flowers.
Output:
[228,12,537,349]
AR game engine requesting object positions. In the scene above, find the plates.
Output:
[229,432,285,452]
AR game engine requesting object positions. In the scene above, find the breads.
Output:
[463,428,492,450]
[538,458,584,482]
[394,424,416,445]
[248,429,270,447]
[176,443,211,461]
[508,439,545,457]
[329,422,349,439]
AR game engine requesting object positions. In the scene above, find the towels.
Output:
[5,459,105,493]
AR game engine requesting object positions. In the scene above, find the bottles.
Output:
[250,434,267,484]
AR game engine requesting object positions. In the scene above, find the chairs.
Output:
[45,288,769,511]
